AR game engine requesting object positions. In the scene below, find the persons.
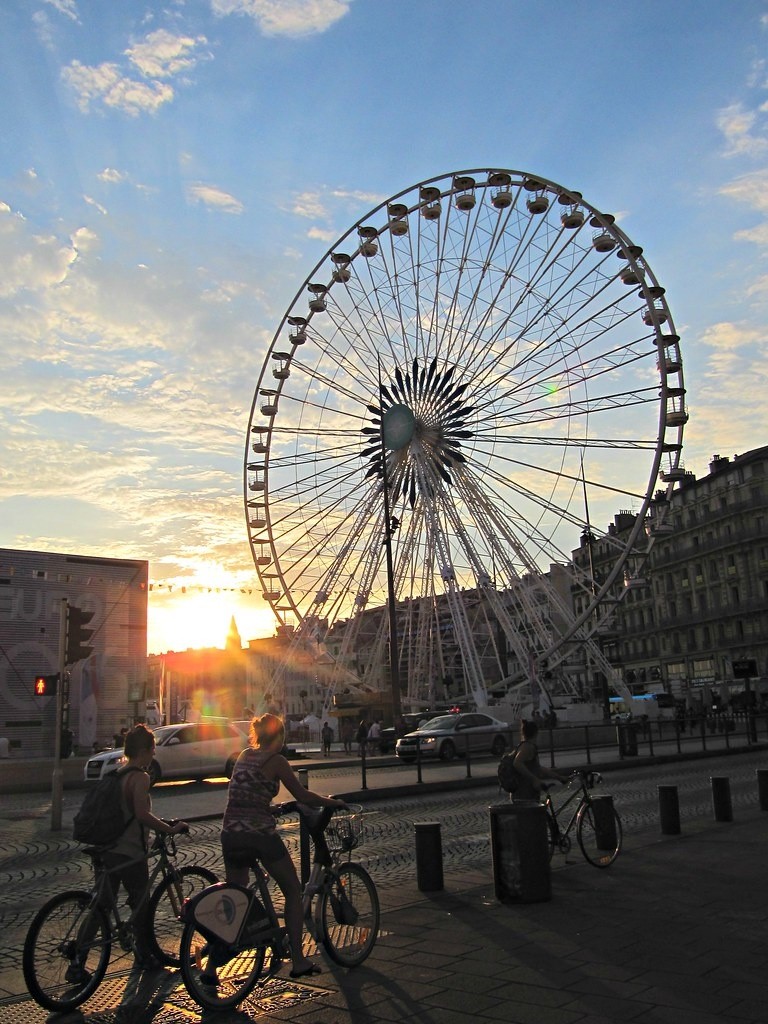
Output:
[65,724,189,987]
[321,715,429,759]
[510,706,569,805]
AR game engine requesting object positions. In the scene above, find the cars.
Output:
[396,713,513,763]
[83,722,250,790]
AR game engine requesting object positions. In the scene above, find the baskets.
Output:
[309,803,364,853]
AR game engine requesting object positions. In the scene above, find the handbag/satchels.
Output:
[320,742,324,751]
[355,731,361,742]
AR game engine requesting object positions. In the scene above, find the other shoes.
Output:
[133,955,165,970]
[64,966,94,984]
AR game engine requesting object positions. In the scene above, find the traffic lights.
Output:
[34,675,59,696]
[69,607,94,664]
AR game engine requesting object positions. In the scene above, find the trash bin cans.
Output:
[488,801,551,904]
[616,724,638,757]
[298,725,310,743]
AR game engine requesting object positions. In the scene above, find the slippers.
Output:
[290,964,322,978]
[199,973,220,986]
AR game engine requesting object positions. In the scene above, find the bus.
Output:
[609,692,676,731]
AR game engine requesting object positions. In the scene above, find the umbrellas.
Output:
[685,678,768,714]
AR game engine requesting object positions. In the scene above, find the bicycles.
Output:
[513,768,624,869]
[179,793,381,1012]
[22,817,223,1013]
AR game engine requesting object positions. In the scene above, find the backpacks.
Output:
[72,767,146,845]
[497,741,538,793]
